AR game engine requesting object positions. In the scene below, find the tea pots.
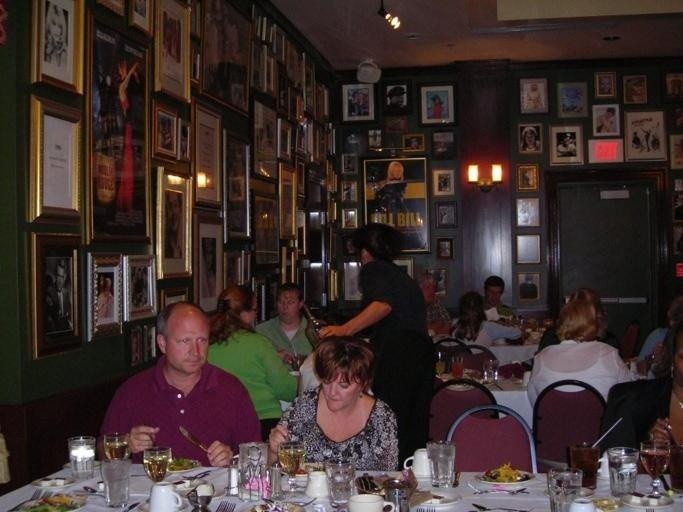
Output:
[403,449,433,478]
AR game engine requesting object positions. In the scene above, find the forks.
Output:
[216,500,236,512]
[467,483,527,494]
[472,503,535,512]
[6,489,53,512]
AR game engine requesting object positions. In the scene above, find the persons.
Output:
[42,1,448,335]
[517,70,683,223]
[417,271,514,340]
[527,287,683,456]
[98,224,436,472]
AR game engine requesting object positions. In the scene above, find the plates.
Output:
[474,471,536,484]
[163,458,202,473]
[409,491,462,507]
[30,477,75,490]
[622,494,673,508]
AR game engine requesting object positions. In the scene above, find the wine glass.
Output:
[640,442,670,498]
[278,442,304,497]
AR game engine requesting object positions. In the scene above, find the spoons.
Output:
[84,486,108,494]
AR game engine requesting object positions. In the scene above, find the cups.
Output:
[325,458,354,503]
[606,449,638,497]
[348,495,395,512]
[426,442,456,488]
[65,425,269,512]
[306,471,328,498]
[569,444,596,489]
[547,469,581,512]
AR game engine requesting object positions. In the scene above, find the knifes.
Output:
[472,491,529,498]
[177,426,209,453]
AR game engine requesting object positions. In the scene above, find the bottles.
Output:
[226,456,238,496]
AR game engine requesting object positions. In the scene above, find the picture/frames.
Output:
[512,68,682,300]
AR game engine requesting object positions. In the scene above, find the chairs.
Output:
[426,336,608,471]
